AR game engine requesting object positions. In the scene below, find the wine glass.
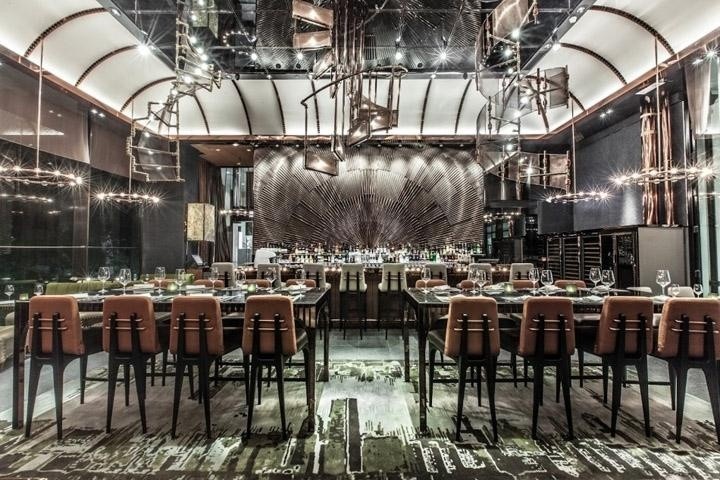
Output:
[4,284,15,302]
[97,266,307,297]
[420,266,703,299]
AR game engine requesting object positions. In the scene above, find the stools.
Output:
[24,294,105,441]
[502,297,577,444]
[102,292,169,436]
[577,294,656,440]
[653,296,720,446]
[426,295,502,445]
[24,276,316,406]
[211,261,540,343]
[409,278,593,389]
[240,294,309,440]
[167,295,248,443]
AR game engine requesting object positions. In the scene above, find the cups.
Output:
[33,284,43,296]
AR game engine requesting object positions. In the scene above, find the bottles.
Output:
[289,241,482,263]
